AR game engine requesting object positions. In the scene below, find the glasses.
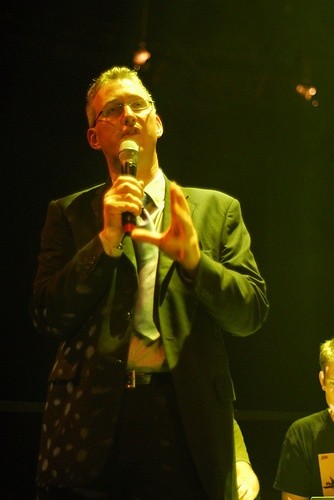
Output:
[92,99,155,126]
[324,379,334,388]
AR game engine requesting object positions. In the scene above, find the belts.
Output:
[124,369,171,388]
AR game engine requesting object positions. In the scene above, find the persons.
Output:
[33,67,269,500]
[273,338,334,500]
[233,418,260,500]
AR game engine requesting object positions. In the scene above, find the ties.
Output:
[131,197,160,341]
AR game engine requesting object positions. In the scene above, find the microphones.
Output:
[117,140,140,237]
